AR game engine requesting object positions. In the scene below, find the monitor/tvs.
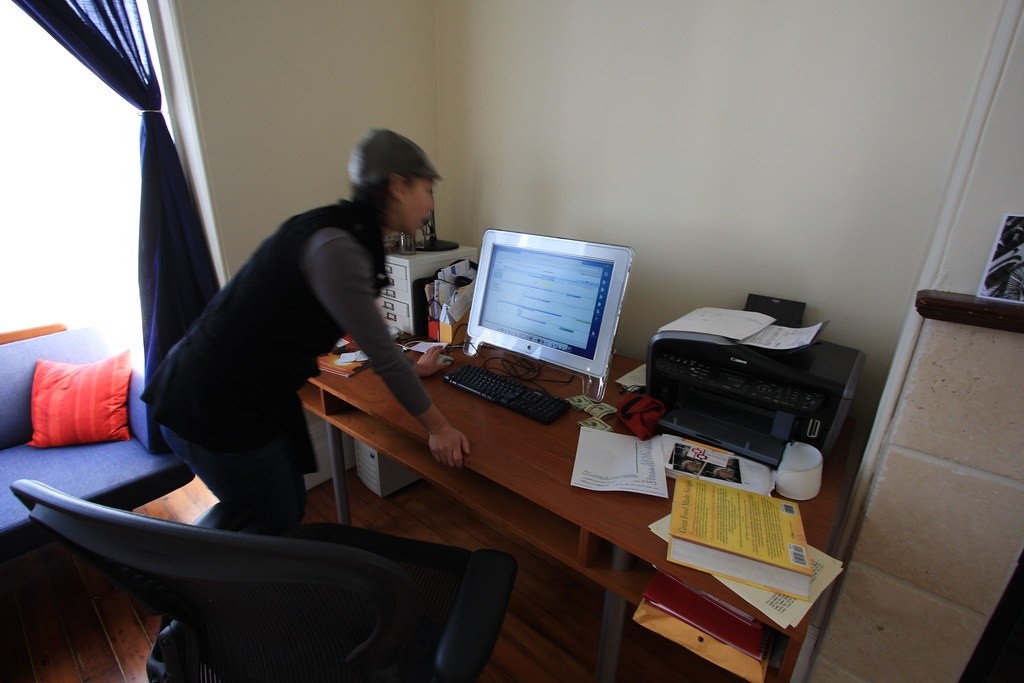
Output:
[461,227,634,403]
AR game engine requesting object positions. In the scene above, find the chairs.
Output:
[10,478,518,683]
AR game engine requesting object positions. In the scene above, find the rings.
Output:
[424,351,428,354]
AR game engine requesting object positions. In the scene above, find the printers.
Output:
[643,293,867,468]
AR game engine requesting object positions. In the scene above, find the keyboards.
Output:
[443,362,571,425]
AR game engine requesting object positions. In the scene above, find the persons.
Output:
[681,460,735,482]
[140,129,469,683]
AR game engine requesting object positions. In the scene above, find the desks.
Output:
[299,335,855,682]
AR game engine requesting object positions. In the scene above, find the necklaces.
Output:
[428,421,449,435]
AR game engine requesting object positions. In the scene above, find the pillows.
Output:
[26,350,134,448]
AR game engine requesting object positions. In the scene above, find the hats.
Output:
[350,129,442,187]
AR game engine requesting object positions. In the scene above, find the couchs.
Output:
[0,323,195,569]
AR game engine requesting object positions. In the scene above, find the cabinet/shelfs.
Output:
[372,244,480,336]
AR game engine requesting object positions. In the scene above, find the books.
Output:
[316,348,373,377]
[666,472,813,602]
[644,572,773,661]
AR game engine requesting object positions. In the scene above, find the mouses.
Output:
[435,354,455,366]
